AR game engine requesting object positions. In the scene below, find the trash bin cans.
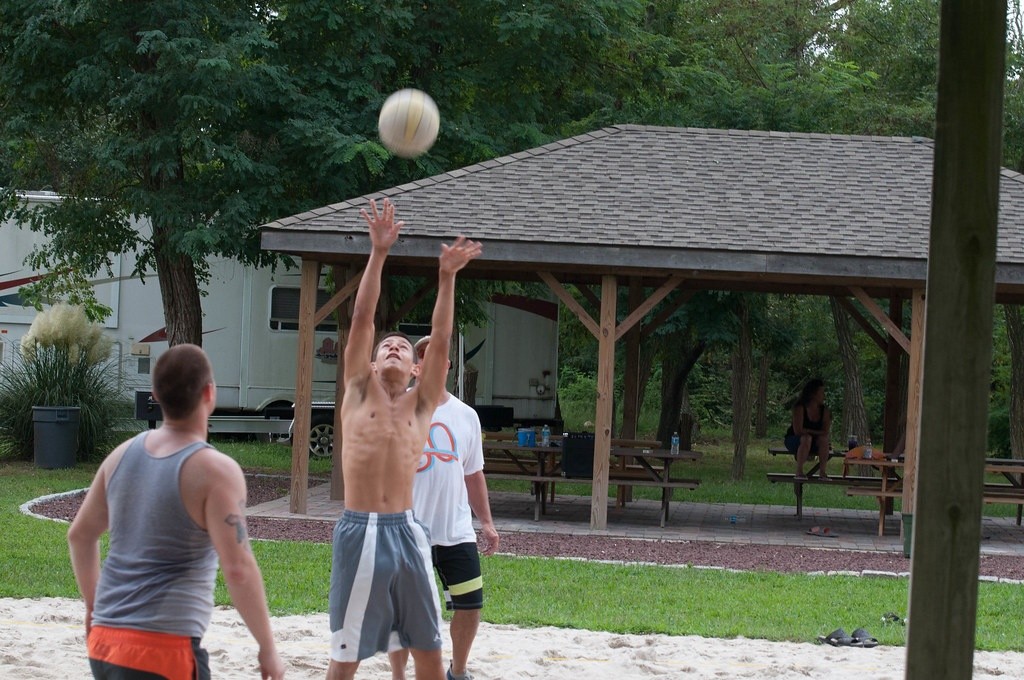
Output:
[517,428,535,447]
[32,405,82,469]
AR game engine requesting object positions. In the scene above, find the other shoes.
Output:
[447,668,474,680]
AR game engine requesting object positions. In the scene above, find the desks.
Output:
[842,458,1024,537]
[483,442,704,528]
[486,430,661,506]
[768,446,1024,525]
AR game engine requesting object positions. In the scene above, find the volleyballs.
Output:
[377,88,440,157]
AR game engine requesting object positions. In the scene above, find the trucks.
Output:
[0,187,565,462]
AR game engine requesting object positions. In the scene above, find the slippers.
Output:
[806,525,839,537]
[850,628,879,646]
[819,628,863,646]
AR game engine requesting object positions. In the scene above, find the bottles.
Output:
[848,437,857,451]
[670,431,680,455]
[724,516,746,523]
[864,438,872,459]
[542,425,550,448]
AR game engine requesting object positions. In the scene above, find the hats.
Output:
[414,335,431,348]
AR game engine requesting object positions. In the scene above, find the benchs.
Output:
[766,473,1024,504]
[483,457,702,528]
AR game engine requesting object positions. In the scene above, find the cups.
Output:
[517,429,536,447]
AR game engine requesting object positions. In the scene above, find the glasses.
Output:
[416,349,426,359]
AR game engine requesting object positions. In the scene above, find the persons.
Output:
[785,379,832,481]
[389,336,498,680]
[66,344,286,680]
[887,410,906,461]
[325,199,483,680]
